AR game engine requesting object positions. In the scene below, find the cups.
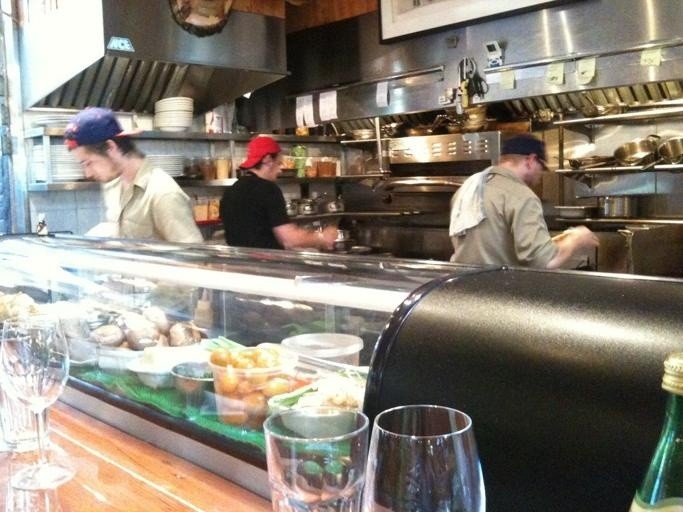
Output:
[1,329,51,452]
[282,144,341,177]
[264,409,368,511]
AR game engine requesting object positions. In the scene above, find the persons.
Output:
[63,108,204,244]
[447,134,601,270]
[219,136,338,251]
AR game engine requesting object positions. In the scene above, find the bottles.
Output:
[625,349,683,512]
[187,155,230,179]
[192,195,222,221]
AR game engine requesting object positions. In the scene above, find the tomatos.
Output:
[210,347,292,428]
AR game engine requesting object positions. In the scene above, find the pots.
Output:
[283,192,347,216]
[553,133,682,169]
[403,114,446,136]
[575,194,639,219]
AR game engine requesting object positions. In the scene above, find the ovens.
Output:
[385,130,520,194]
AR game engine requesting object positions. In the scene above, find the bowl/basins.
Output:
[168,362,215,396]
[267,372,367,445]
[381,122,409,138]
[127,349,210,389]
[349,128,377,139]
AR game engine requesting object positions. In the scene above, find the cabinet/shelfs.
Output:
[553,112,682,226]
[23,125,389,193]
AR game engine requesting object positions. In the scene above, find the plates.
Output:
[27,115,87,183]
[153,96,195,133]
[145,154,186,179]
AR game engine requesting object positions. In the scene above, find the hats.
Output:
[64,106,145,148]
[239,137,281,169]
[502,134,550,173]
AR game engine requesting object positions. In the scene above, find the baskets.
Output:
[315,161,337,177]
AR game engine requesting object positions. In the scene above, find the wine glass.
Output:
[1,314,76,490]
[363,405,487,512]
